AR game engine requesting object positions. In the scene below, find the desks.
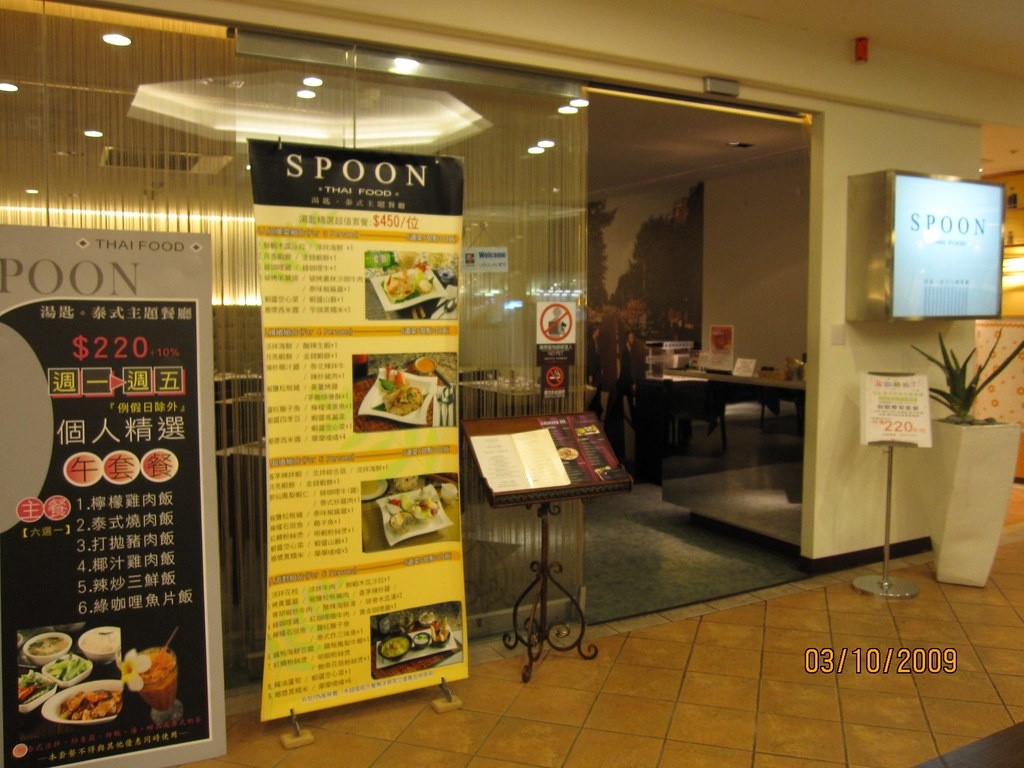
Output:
[460,376,597,412]
[645,359,806,453]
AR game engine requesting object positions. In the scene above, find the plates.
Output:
[369,268,447,311]
[358,368,438,425]
[361,480,388,500]
[376,624,457,669]
[377,483,454,546]
[42,679,124,724]
[18,633,24,647]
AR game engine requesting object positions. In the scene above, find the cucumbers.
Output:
[49,651,93,682]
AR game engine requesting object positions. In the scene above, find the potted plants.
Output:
[911,328,1024,587]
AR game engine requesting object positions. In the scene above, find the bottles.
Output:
[352,354,368,379]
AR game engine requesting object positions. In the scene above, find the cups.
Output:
[441,484,457,504]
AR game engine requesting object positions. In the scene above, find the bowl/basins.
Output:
[416,357,437,375]
[413,633,431,647]
[42,653,93,686]
[17,672,57,712]
[78,626,121,665]
[23,632,73,664]
[432,630,450,648]
[378,632,412,660]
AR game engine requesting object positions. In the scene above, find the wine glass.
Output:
[129,646,183,724]
